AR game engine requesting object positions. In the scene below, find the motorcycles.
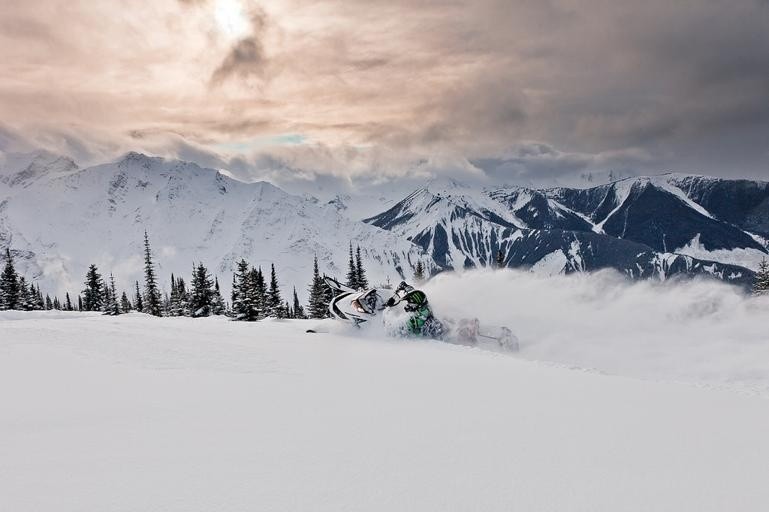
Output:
[312,276,519,355]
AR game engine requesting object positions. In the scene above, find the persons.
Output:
[399,280,443,339]
